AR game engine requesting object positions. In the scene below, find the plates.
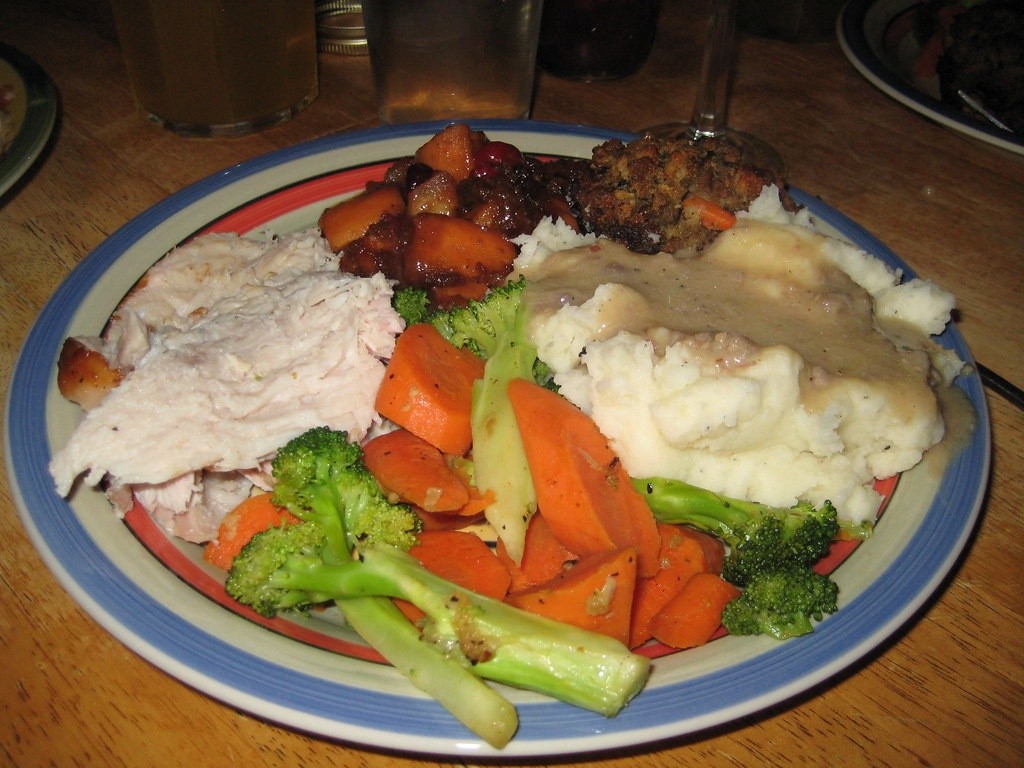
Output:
[4,119,991,757]
[0,42,57,196]
[835,0,1024,156]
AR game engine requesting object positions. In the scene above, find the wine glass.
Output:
[637,0,786,180]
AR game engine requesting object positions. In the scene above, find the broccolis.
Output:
[628,477,841,638]
[394,276,561,570]
[271,424,520,748]
[222,526,651,719]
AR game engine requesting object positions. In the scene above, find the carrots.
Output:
[202,325,733,660]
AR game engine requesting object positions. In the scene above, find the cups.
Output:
[112,0,319,140]
[536,0,660,81]
[361,0,542,124]
